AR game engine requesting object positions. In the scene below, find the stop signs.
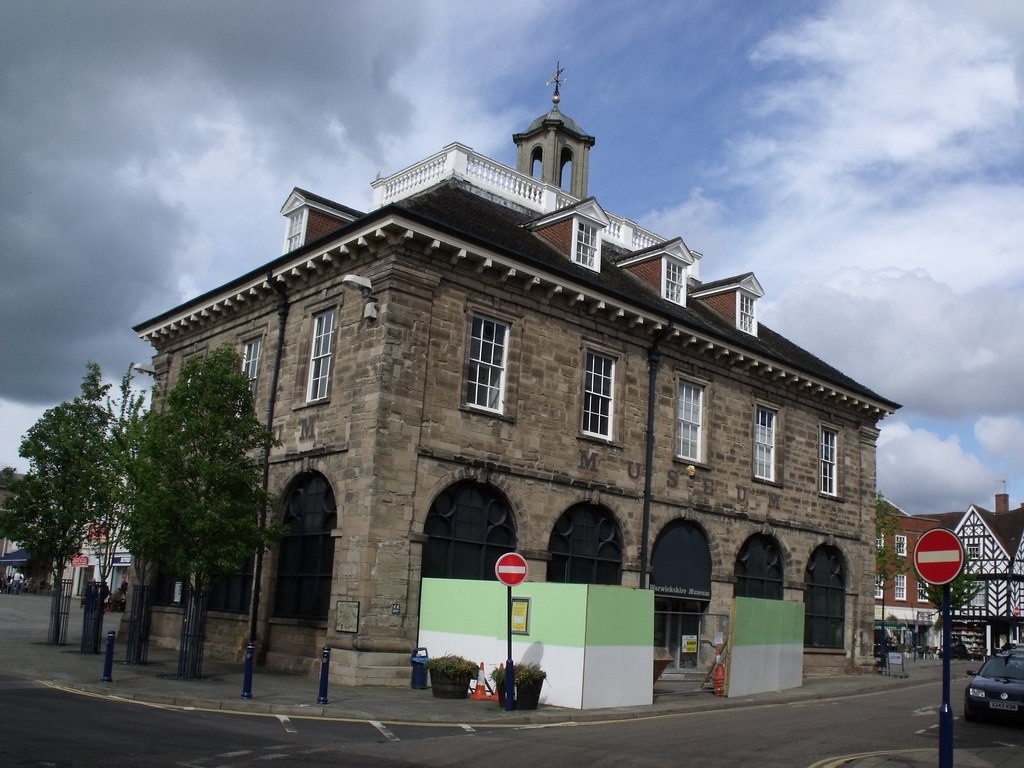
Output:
[913,528,965,585]
[495,552,528,587]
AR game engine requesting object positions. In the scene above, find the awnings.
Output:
[874,621,907,630]
[0,549,32,566]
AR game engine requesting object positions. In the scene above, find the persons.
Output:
[104,579,128,615]
[0,569,25,595]
[878,635,898,657]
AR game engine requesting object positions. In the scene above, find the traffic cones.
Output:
[711,649,725,697]
[471,662,489,701]
[489,663,506,702]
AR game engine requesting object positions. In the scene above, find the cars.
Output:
[963,643,1024,725]
